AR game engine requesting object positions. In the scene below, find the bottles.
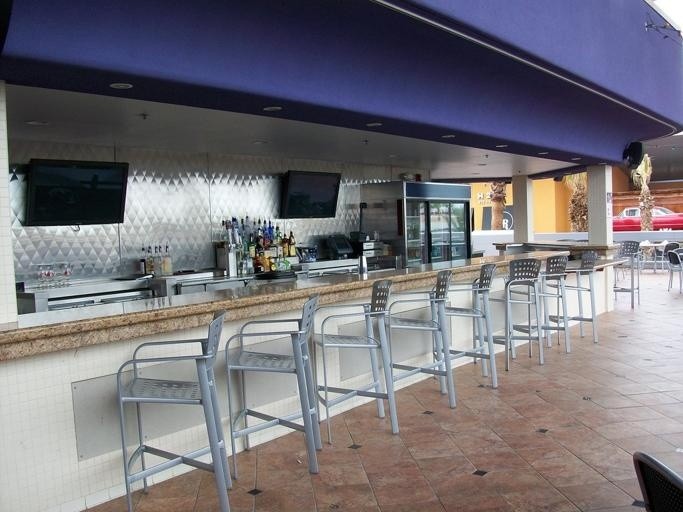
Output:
[219,211,296,278]
[138,241,172,276]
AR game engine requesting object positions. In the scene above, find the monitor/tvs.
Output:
[280,168,341,219]
[23,157,129,227]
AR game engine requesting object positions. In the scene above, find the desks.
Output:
[638,242,664,275]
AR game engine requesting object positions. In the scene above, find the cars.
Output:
[612,206,682,231]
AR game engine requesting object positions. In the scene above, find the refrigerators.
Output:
[359,180,471,272]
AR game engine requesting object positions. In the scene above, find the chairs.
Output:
[632,450,683,512]
[311,278,399,445]
[473,258,544,372]
[613,241,642,309]
[384,268,456,410]
[429,263,499,395]
[527,254,569,361]
[556,250,598,347]
[117,310,232,511]
[224,292,323,480]
[660,241,683,294]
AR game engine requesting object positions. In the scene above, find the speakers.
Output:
[624,141,643,169]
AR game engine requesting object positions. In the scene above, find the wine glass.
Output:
[36,263,72,290]
